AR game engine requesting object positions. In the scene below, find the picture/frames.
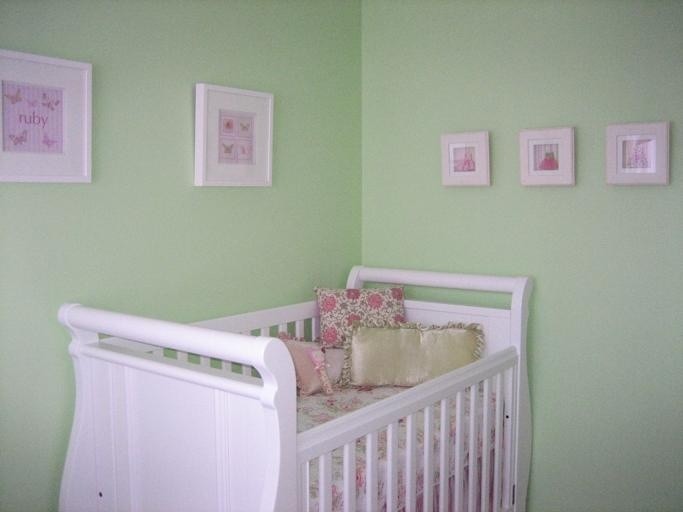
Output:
[193,82,273,189]
[438,119,670,190]
[0,47,94,184]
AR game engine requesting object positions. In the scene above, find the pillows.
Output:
[266,284,485,399]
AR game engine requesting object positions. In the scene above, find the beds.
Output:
[54,262,537,512]
